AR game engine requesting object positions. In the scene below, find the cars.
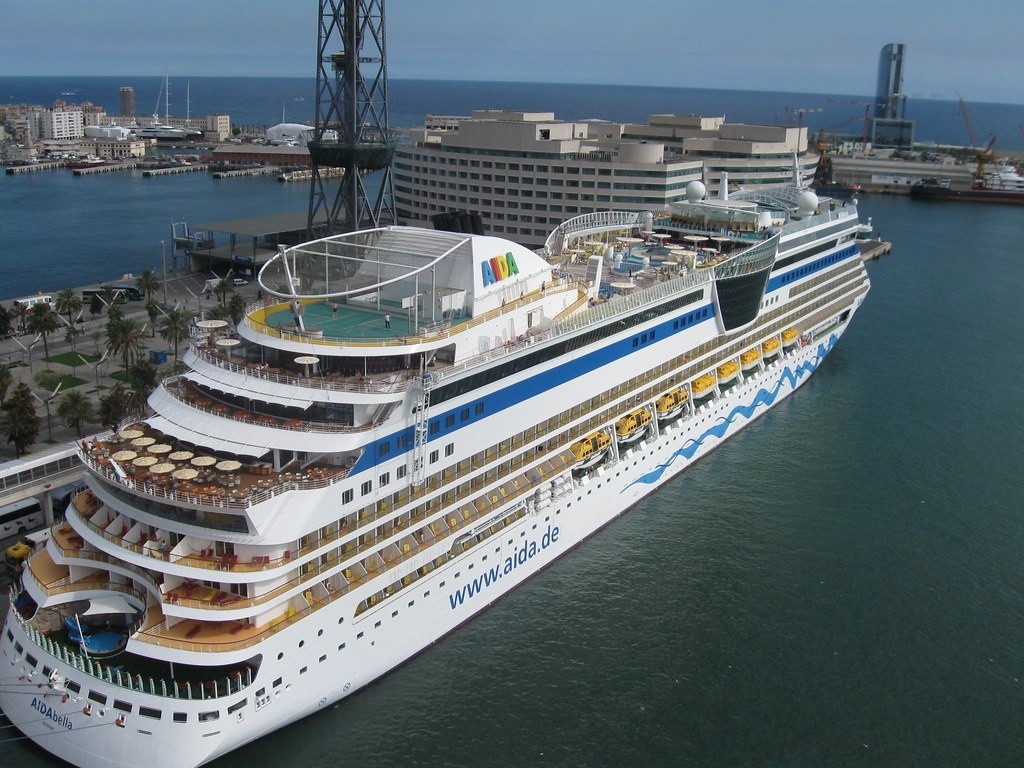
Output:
[233,279,248,286]
[259,138,299,147]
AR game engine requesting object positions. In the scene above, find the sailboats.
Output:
[135,65,187,140]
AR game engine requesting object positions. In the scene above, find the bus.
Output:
[82,288,130,305]
[14,295,53,311]
[100,282,145,301]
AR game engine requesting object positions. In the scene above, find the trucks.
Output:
[4,524,60,579]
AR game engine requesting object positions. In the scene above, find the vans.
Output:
[205,279,222,293]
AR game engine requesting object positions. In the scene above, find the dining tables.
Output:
[222,476,239,482]
[92,450,107,455]
[313,469,322,475]
[198,472,210,478]
[179,390,301,429]
[178,484,193,492]
[234,492,248,497]
[258,482,272,487]
[205,487,220,495]
[112,434,123,439]
[284,475,295,480]
[156,479,171,485]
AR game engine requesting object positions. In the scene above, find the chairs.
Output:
[269,366,668,639]
[185,624,201,638]
[77,493,291,571]
[57,524,242,607]
[231,621,246,635]
[82,434,336,502]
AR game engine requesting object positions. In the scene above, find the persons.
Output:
[161,540,167,551]
[298,370,303,377]
[332,300,339,318]
[87,441,94,453]
[255,361,269,372]
[113,423,119,434]
[168,476,176,493]
[290,301,305,332]
[207,336,213,348]
[153,474,158,481]
[196,339,203,347]
[385,313,391,329]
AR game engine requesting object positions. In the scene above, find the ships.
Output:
[0,148,872,768]
